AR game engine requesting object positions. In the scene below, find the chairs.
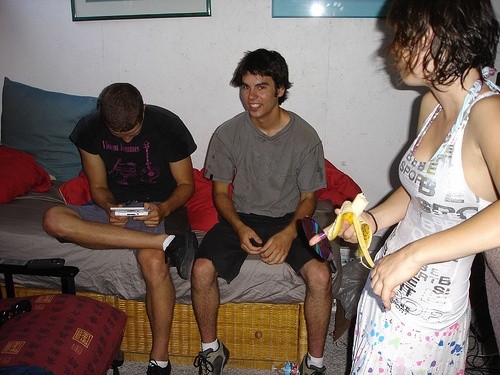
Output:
[0,258,128,375]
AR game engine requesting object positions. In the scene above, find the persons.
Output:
[308,0,500,375]
[190,50,332,375]
[43,83,198,375]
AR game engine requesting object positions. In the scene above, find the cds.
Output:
[302,216,334,261]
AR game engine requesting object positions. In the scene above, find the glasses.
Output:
[0,300,32,326]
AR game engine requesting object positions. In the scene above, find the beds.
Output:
[0,144,362,369]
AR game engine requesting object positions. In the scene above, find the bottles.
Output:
[271,362,300,374]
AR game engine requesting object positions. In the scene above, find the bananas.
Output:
[327,194,375,269]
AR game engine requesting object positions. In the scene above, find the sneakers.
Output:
[165,231,198,280]
[193,339,230,375]
[146,358,171,375]
[298,352,326,375]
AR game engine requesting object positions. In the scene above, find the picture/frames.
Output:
[71,0,211,21]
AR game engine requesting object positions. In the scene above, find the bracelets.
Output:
[363,211,378,235]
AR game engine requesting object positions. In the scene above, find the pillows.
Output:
[1,76,98,180]
